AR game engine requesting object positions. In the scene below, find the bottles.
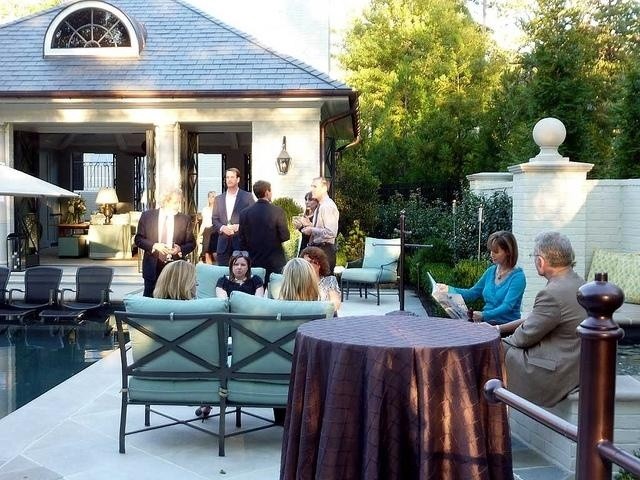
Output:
[326,302,334,319]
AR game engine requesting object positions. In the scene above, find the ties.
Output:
[314,204,320,227]
[159,216,168,263]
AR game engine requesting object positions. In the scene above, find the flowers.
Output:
[68,192,86,216]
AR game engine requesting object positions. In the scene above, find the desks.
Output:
[280,315,515,480]
[88,225,132,260]
[57,223,88,237]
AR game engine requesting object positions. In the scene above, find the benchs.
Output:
[191,262,285,298]
[113,291,334,455]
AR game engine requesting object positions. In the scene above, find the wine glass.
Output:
[163,242,174,261]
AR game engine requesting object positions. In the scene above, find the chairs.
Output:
[341,237,402,305]
[61,265,111,311]
[0,267,11,308]
[8,266,63,311]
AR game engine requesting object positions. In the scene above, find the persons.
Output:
[216,251,265,356]
[213,167,254,266]
[293,192,319,258]
[299,247,342,316]
[273,259,322,426]
[239,180,290,289]
[135,187,196,298]
[199,192,219,264]
[299,177,340,275]
[437,231,590,404]
[153,261,210,424]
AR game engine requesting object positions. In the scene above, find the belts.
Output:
[316,242,328,247]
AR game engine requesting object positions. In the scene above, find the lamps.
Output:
[95,187,119,224]
[277,136,291,174]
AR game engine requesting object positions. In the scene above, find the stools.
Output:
[39,310,87,324]
[58,237,81,259]
[0,309,37,325]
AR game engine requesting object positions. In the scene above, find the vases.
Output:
[75,213,79,224]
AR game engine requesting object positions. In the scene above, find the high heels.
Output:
[195,405,213,423]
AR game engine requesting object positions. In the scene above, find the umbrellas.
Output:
[0,163,80,197]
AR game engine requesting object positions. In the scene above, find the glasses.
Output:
[529,253,546,262]
[233,251,249,258]
